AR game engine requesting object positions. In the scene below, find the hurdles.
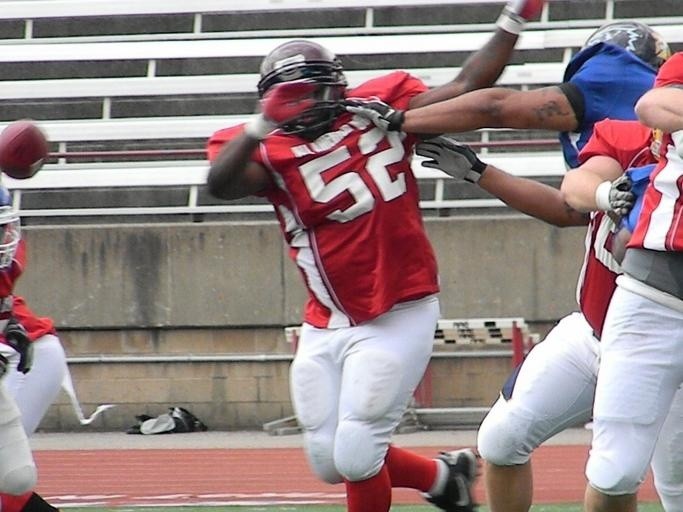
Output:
[415,316,541,426]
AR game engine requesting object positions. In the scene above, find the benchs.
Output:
[0,0,683,221]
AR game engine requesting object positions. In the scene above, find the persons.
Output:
[335,21,683,511]
[0,292,68,444]
[0,180,39,511]
[206,1,542,511]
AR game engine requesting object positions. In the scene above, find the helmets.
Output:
[581,23,672,72]
[0,186,21,269]
[258,40,349,136]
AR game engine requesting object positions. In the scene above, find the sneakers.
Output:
[419,448,482,511]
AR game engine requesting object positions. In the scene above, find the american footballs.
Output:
[0,123,47,179]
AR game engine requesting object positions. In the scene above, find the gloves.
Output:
[245,82,318,141]
[414,136,487,184]
[4,318,32,374]
[496,0,545,37]
[596,176,636,218]
[345,96,409,132]
